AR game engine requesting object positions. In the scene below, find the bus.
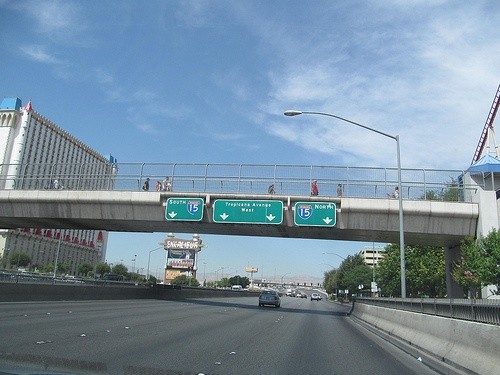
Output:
[232,285,243,292]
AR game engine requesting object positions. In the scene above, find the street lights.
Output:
[322,253,348,301]
[318,263,338,302]
[284,110,406,298]
[147,244,167,282]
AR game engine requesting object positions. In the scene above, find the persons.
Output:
[156,176,173,192]
[337,184,344,197]
[268,184,276,195]
[142,178,150,191]
[44,176,65,190]
[311,180,319,196]
[393,186,400,199]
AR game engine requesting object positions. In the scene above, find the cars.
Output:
[259,291,281,308]
[100,274,124,281]
[286,289,322,301]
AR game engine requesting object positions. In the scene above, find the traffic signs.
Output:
[212,199,283,225]
[294,202,337,228]
[165,197,204,222]
